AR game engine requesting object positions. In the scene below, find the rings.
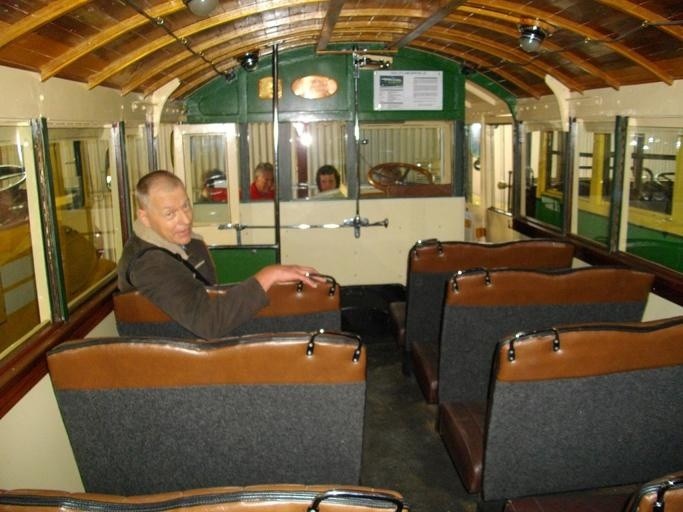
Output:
[304,271,309,277]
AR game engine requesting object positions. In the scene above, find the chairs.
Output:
[578,178,610,195]
[629,200,667,213]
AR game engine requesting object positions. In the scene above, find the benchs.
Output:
[501,470,682,512]
[436,316,683,512]
[409,263,656,406]
[0,484,405,512]
[111,275,342,340]
[45,330,367,499]
[390,238,575,377]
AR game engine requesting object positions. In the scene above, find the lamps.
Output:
[234,48,260,72]
[517,17,555,54]
[181,0,219,18]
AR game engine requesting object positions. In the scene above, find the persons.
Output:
[201,170,226,202]
[250,162,274,199]
[114,169,328,343]
[316,165,340,192]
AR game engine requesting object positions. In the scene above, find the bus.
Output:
[534,127,683,272]
